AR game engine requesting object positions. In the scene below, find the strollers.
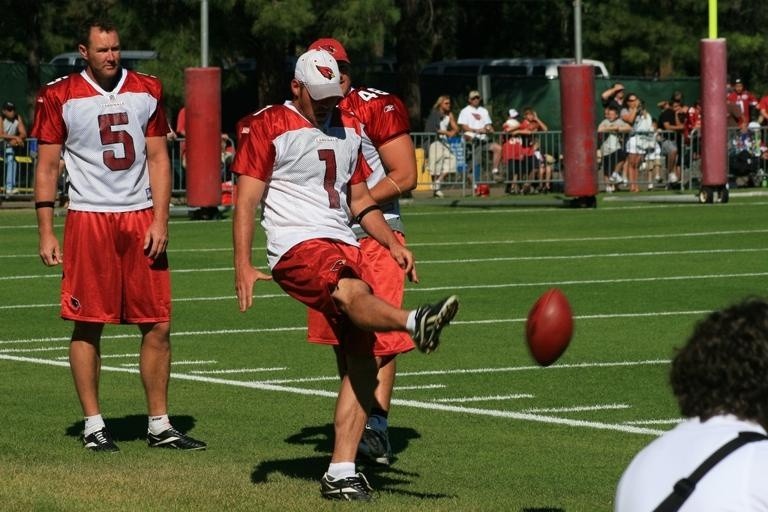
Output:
[726,111,762,187]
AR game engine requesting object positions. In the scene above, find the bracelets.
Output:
[355,205,383,224]
[385,176,402,195]
[35,201,55,209]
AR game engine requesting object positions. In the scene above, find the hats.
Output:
[308,36,352,65]
[3,100,15,109]
[469,90,481,99]
[293,48,346,101]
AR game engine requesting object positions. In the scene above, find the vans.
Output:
[53,51,157,72]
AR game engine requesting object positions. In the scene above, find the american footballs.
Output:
[527,288,573,366]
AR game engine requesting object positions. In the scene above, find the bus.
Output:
[374,57,609,80]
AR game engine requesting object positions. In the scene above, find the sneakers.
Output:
[146,427,207,452]
[81,426,121,453]
[510,183,552,195]
[647,172,678,190]
[605,185,615,192]
[492,171,506,183]
[357,427,393,466]
[609,172,622,183]
[6,189,19,199]
[432,181,443,199]
[411,294,460,356]
[320,472,379,503]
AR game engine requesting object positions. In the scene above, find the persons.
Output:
[612,295,767,511]
[307,37,418,467]
[227,46,460,501]
[0,101,189,195]
[31,14,207,452]
